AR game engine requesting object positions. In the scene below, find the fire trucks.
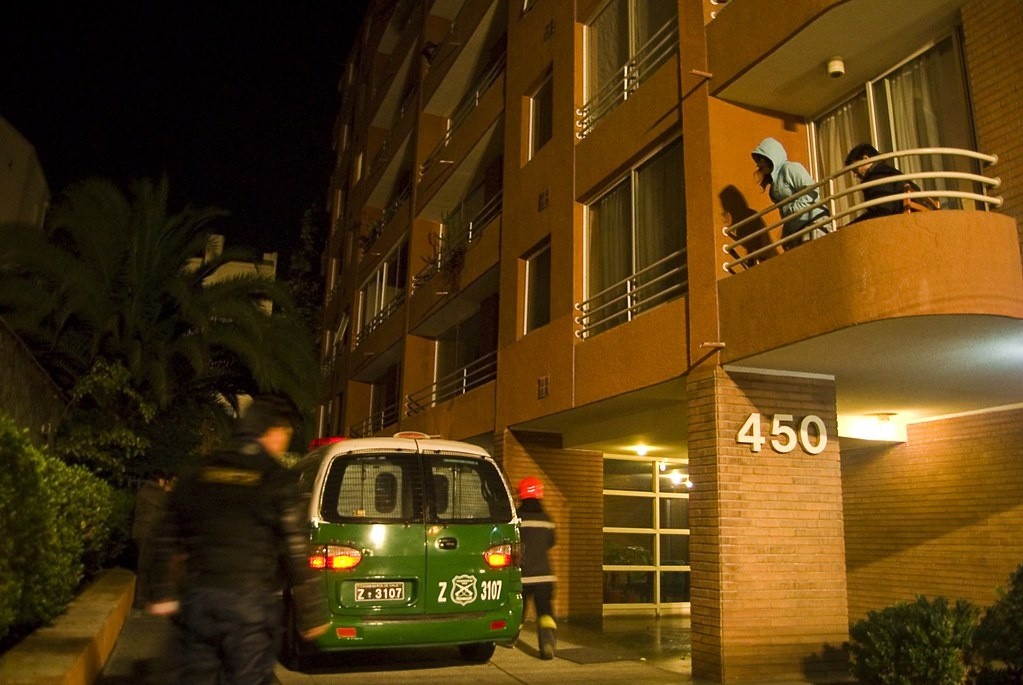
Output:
[276,428,523,666]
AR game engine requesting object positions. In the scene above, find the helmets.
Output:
[517,477,543,499]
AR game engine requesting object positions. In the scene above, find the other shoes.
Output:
[542,644,554,660]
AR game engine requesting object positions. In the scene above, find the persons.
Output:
[132,474,170,615]
[500,476,556,658]
[751,138,832,245]
[151,402,331,685]
[838,143,922,229]
